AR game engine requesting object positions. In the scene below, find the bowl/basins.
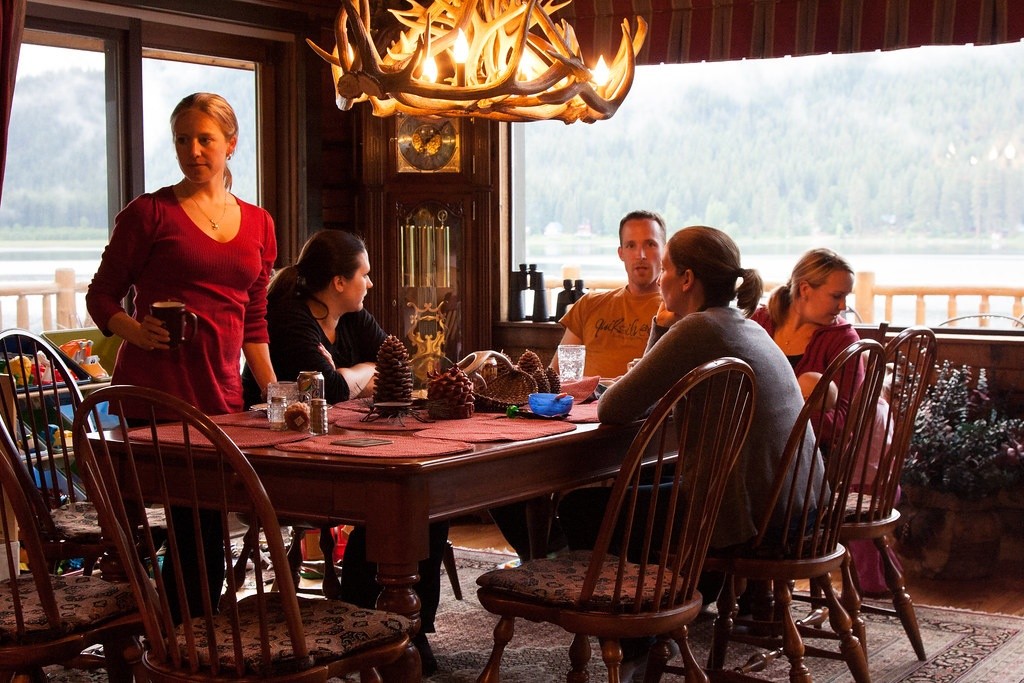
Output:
[527,392,574,419]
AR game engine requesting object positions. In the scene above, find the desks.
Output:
[70,398,725,683]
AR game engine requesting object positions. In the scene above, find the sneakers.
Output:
[223,542,254,568]
[259,542,291,563]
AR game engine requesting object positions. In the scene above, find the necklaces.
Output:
[182,180,226,230]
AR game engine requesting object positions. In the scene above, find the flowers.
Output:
[881,340,1024,488]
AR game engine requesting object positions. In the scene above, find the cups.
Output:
[266,380,312,432]
[557,344,587,381]
[148,301,198,349]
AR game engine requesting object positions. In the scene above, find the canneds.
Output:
[297,371,324,404]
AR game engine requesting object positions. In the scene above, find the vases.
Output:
[860,470,952,567]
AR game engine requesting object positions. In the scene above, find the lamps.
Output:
[306,0,648,125]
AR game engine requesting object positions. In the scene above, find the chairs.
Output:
[1,327,939,683]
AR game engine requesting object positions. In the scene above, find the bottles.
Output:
[270,396,288,432]
[309,398,328,435]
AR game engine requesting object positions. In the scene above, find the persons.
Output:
[235,230,449,683]
[557,226,834,683]
[86,93,279,652]
[487,212,683,569]
[640,257,903,637]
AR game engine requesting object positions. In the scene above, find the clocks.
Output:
[392,100,463,173]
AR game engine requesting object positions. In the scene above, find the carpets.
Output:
[0,539,1023,683]
